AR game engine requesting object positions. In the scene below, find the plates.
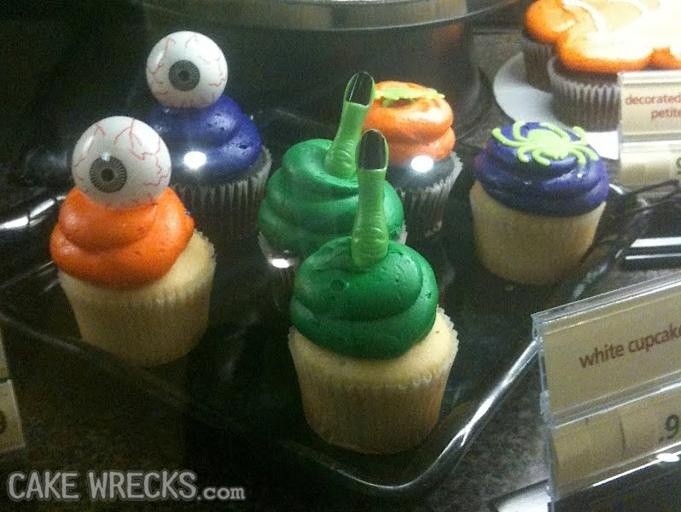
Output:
[1,99,653,504]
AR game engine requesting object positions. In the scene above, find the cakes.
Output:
[650,8,681,78]
[45,114,216,363]
[517,1,606,88]
[363,78,463,247]
[256,68,410,281]
[468,119,610,288]
[135,28,273,245]
[546,18,654,134]
[285,129,462,455]
